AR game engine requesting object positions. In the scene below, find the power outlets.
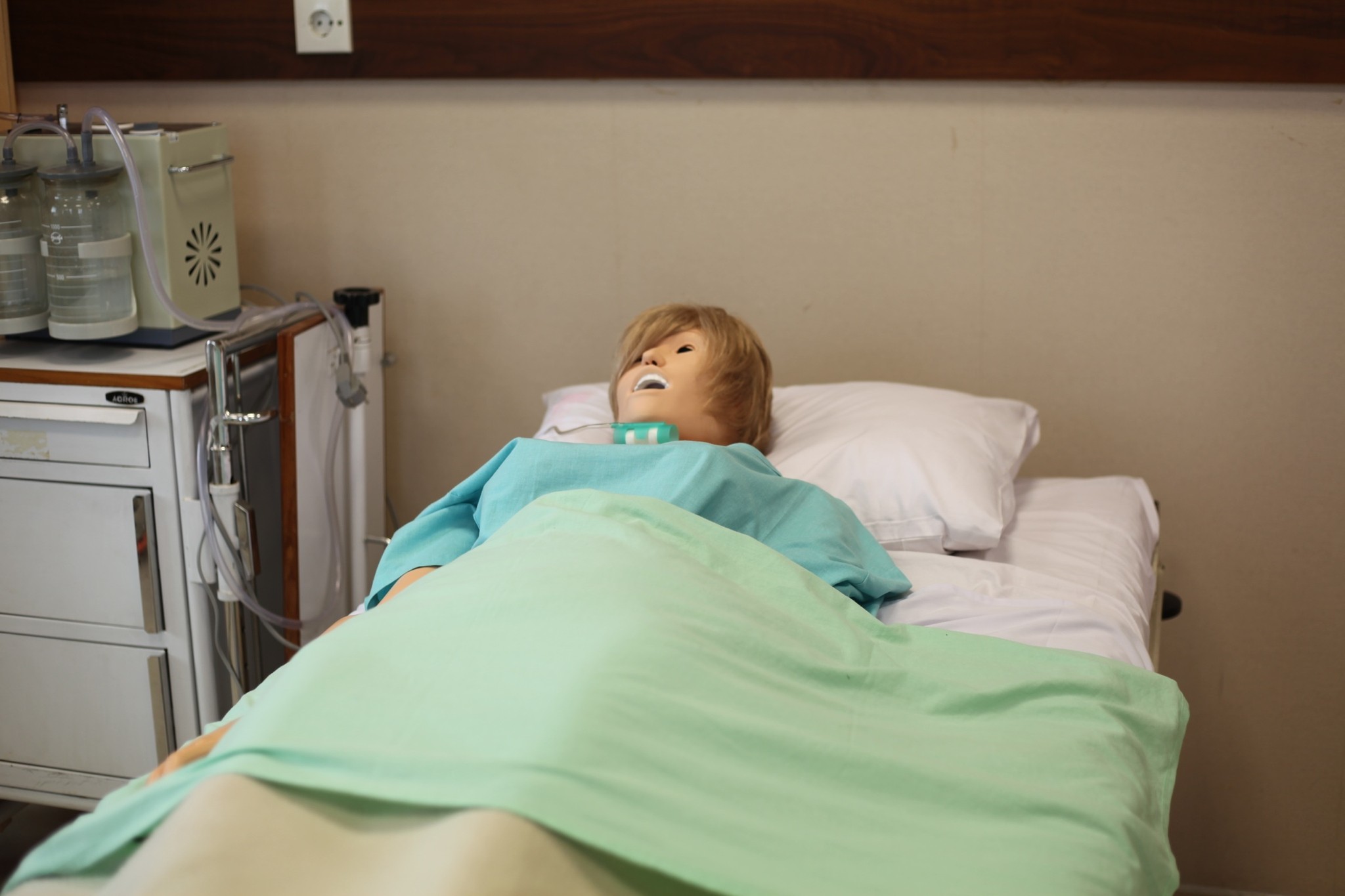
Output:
[293,3,351,53]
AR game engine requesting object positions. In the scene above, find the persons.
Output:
[365,296,899,677]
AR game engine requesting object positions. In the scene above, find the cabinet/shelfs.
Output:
[0,357,279,814]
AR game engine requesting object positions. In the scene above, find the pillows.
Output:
[536,382,1038,550]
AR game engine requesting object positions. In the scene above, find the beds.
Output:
[1,477,1188,896]
